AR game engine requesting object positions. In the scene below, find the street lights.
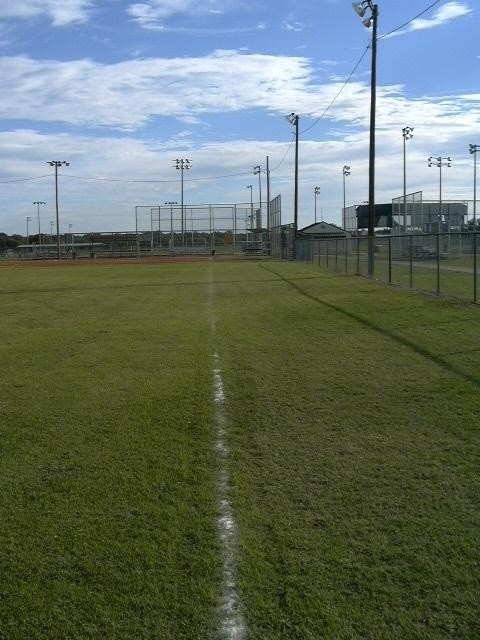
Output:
[172,155,193,256]
[25,159,74,261]
[427,156,452,231]
[285,112,298,239]
[314,185,321,225]
[247,184,253,241]
[402,125,413,231]
[253,166,261,220]
[352,1,379,278]
[341,164,350,230]
[164,200,177,254]
[468,143,480,228]
